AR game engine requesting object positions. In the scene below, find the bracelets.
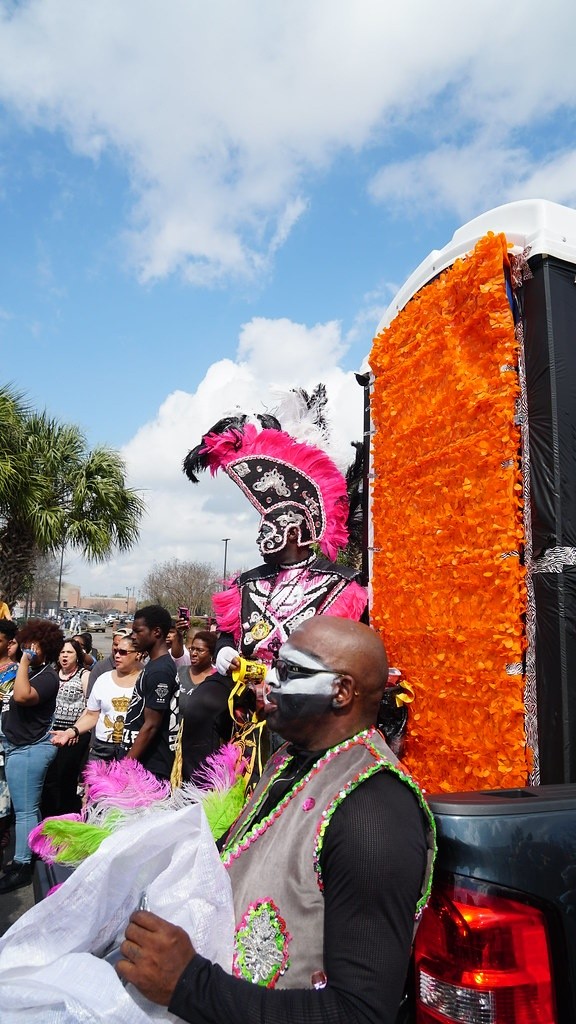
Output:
[70,727,79,738]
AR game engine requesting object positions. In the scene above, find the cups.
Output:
[231,656,267,685]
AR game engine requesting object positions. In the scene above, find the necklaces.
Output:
[29,664,49,681]
[251,556,309,638]
[59,666,77,688]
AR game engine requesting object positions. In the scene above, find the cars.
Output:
[61,607,132,633]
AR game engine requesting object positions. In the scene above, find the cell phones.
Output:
[177,607,190,630]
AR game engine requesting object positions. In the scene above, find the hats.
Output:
[182,385,350,563]
[112,628,133,638]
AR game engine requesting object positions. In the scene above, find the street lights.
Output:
[221,538,231,592]
[126,587,131,615]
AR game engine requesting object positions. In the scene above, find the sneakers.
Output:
[0,860,33,894]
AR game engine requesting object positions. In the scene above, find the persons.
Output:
[114,615,437,1023]
[1,592,233,870]
[1,621,65,894]
[214,504,370,788]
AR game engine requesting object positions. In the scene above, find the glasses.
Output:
[7,640,17,646]
[270,658,348,682]
[113,648,139,656]
[187,647,210,655]
